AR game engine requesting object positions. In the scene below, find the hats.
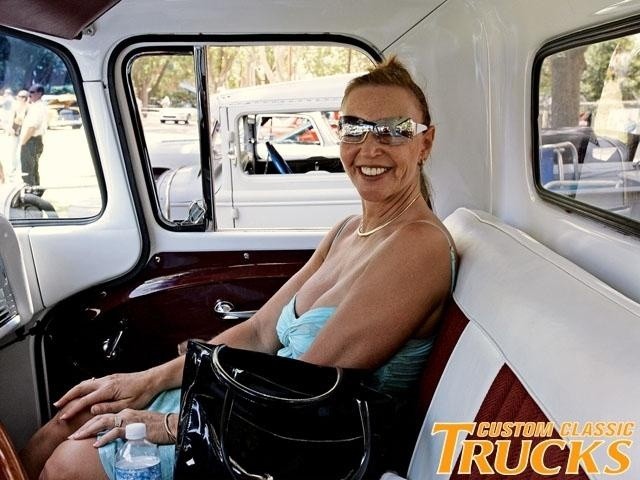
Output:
[17,90,28,97]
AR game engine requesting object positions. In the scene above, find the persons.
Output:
[18,51,459,480]
[18,82,47,187]
[1,88,30,182]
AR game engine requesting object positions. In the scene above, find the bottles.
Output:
[110,422,161,480]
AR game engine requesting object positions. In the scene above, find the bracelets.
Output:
[164,412,176,443]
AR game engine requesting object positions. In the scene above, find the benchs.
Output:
[376,207,640,480]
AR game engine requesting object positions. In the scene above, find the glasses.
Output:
[336,115,427,145]
[30,90,36,94]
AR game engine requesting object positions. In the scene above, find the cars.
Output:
[151,74,639,231]
[159,108,193,124]
[45,107,82,130]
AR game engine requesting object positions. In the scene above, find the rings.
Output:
[114,416,122,427]
[92,376,95,382]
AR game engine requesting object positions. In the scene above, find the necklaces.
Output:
[356,191,422,238]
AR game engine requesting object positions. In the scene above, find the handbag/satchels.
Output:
[172,340,381,480]
